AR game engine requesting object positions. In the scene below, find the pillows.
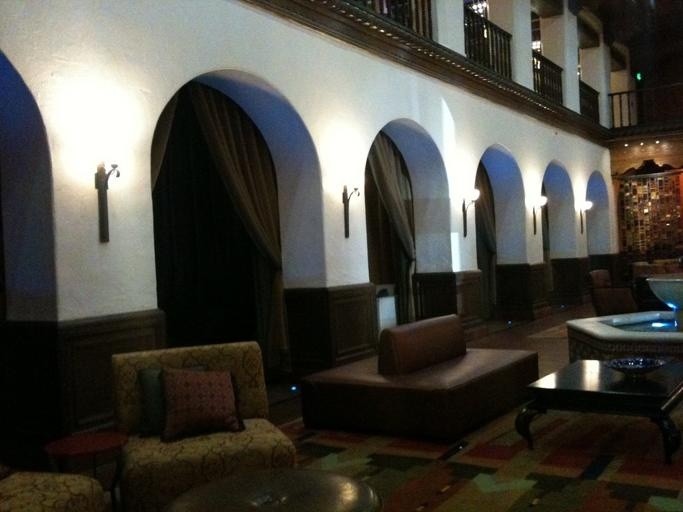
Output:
[131,366,245,437]
[159,362,247,444]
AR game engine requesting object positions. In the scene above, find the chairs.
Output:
[108,336,298,512]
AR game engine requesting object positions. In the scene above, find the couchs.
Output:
[584,257,683,318]
[309,313,539,444]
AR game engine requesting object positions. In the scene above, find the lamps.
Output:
[338,172,598,243]
[93,155,122,243]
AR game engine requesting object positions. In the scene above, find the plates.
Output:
[601,357,667,379]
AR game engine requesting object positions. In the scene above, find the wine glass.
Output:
[645,274,683,329]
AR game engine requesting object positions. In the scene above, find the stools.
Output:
[0,467,106,512]
